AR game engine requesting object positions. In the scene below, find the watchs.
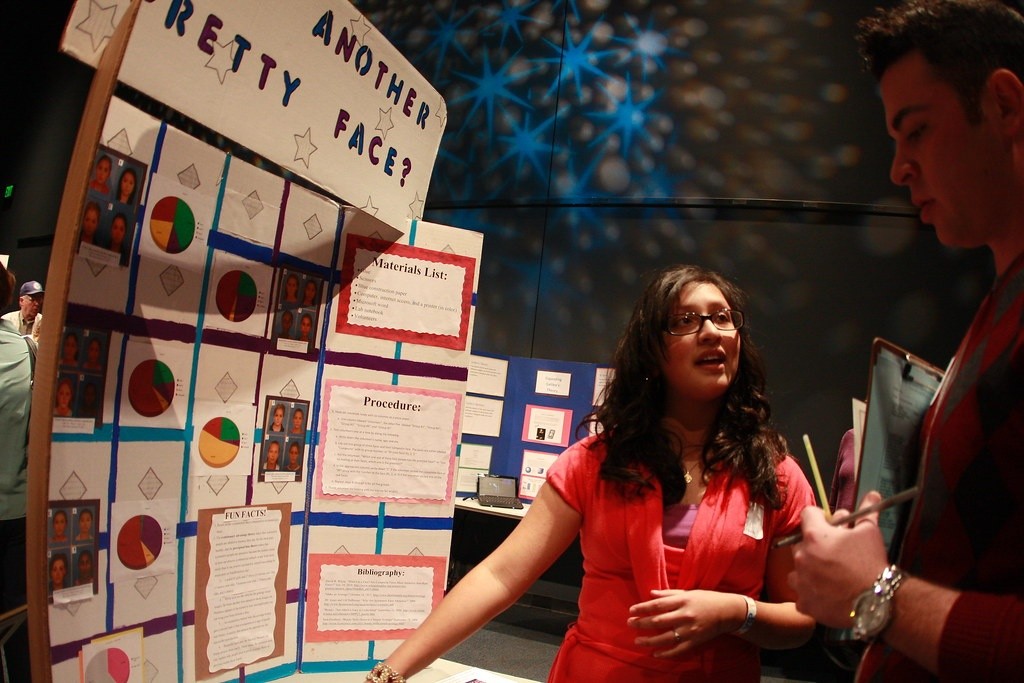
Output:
[849,564,909,642]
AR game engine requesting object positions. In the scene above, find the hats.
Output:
[19,280,45,294]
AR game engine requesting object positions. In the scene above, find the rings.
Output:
[672,628,681,642]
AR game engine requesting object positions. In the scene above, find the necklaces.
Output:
[676,461,703,484]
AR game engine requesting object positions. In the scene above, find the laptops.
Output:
[477,474,523,508]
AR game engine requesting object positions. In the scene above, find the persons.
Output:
[53,332,102,420]
[366,264,821,683]
[0,262,46,683]
[279,273,318,342]
[787,0,1024,683]
[828,427,855,528]
[263,404,304,472]
[48,509,94,590]
[78,155,138,266]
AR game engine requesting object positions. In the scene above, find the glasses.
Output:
[27,297,43,304]
[661,309,744,335]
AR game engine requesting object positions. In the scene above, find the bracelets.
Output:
[731,595,758,636]
[365,661,409,683]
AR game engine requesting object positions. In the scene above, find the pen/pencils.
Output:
[769,432,921,555]
